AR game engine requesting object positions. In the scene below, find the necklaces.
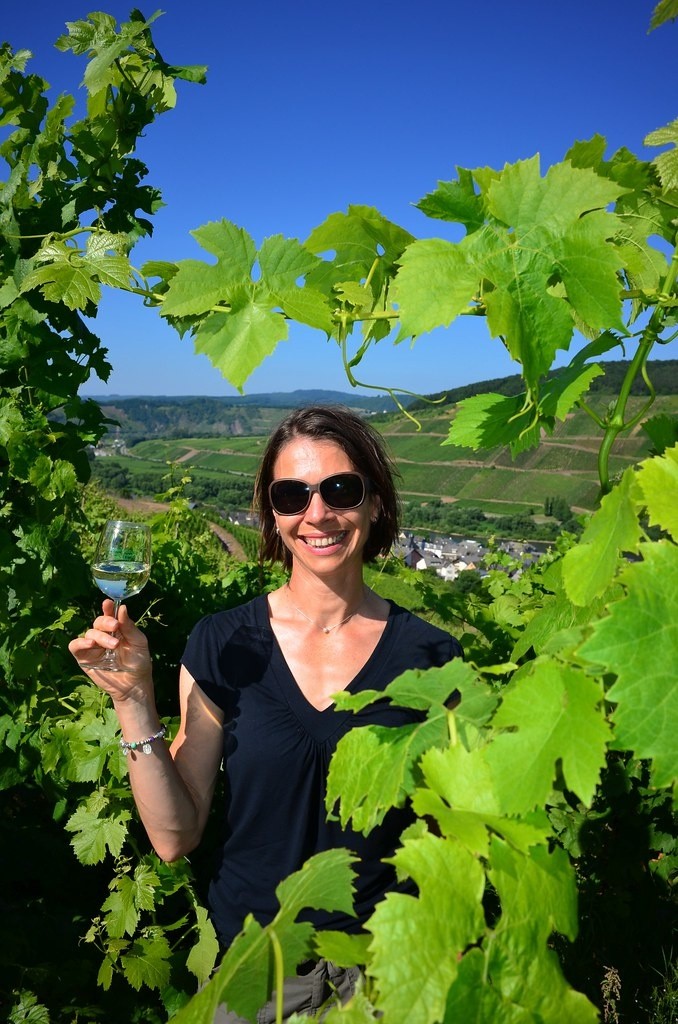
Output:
[284,588,373,633]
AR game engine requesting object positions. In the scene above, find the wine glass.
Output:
[79,519,152,672]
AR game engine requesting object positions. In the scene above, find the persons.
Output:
[69,408,465,1024]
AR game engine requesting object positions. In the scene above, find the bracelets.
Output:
[120,723,166,755]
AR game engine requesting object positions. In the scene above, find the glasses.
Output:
[268,472,366,516]
